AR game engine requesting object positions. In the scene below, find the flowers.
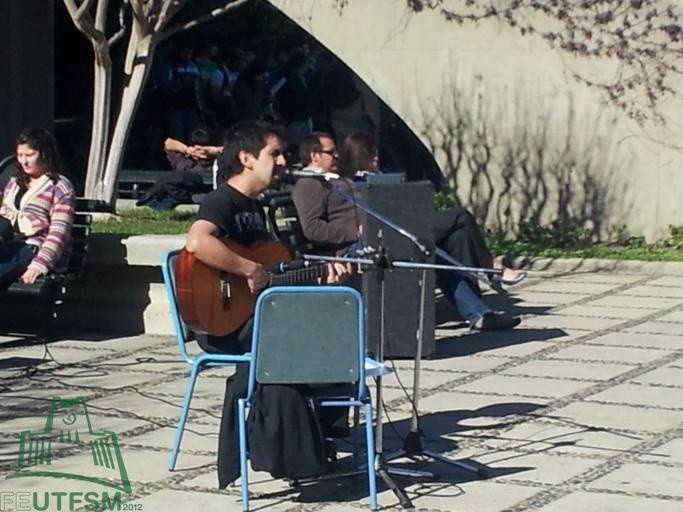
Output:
[280,166,323,184]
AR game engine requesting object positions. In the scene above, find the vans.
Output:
[492,265,527,287]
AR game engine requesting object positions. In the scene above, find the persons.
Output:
[0,128,75,293]
[292,133,519,330]
[164,128,225,176]
[186,123,353,466]
[342,132,526,295]
[144,33,380,128]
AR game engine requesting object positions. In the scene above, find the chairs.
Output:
[161,241,397,512]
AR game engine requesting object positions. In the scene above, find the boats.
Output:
[364,179,437,359]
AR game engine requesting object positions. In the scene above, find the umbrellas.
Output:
[474,312,521,329]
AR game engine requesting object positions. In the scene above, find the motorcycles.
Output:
[291,266,437,508]
[356,269,487,477]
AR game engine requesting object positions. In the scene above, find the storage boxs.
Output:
[0,198,103,342]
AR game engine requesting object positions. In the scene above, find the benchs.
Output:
[0,198,103,342]
